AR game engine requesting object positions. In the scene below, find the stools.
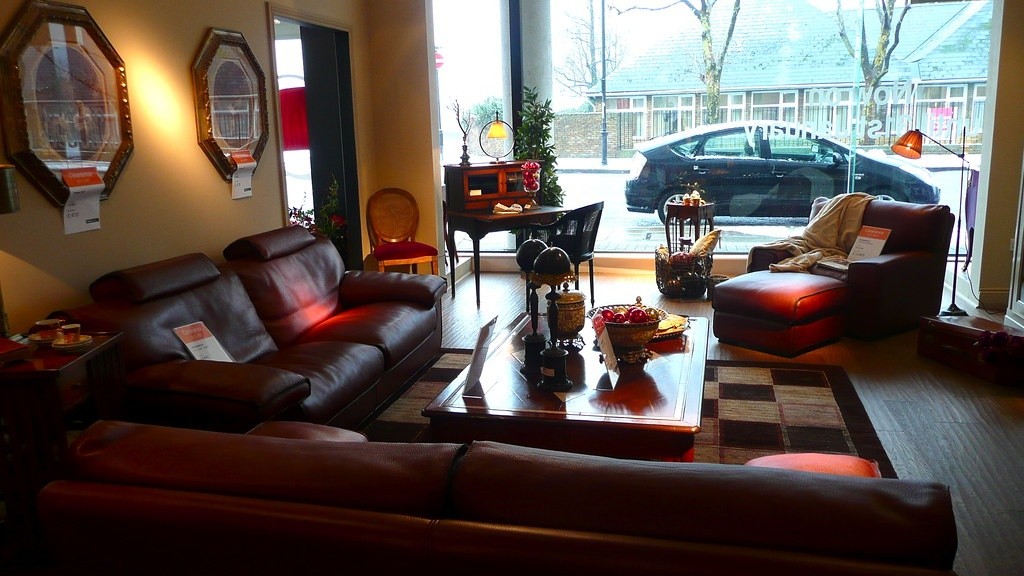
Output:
[713,269,850,358]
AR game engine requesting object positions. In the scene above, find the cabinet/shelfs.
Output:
[916,315,1024,392]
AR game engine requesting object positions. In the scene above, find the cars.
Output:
[624,118,942,227]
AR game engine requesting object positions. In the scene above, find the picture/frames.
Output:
[0,0,134,210]
[191,26,270,182]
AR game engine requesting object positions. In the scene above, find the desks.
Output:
[666,203,715,260]
[443,158,578,312]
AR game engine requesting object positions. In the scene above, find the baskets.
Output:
[654,246,713,298]
[586,304,669,347]
[653,315,691,340]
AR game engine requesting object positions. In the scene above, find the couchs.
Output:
[33,224,960,576]
[745,190,956,343]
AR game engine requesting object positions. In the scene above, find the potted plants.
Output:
[511,85,562,252]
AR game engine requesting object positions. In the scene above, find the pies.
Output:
[655,314,688,335]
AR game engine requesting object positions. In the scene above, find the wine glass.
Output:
[521,168,542,209]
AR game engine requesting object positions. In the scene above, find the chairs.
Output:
[366,185,440,275]
[516,200,605,313]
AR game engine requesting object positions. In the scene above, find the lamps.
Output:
[891,124,971,318]
[0,164,28,343]
[479,111,518,164]
[519,238,581,394]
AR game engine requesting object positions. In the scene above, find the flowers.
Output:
[978,328,1013,351]
[285,178,347,240]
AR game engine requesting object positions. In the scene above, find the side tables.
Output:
[0,326,129,566]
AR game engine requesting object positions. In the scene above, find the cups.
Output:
[684,197,691,205]
[546,282,586,338]
[692,198,700,207]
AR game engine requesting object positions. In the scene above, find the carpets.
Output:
[357,346,903,481]
